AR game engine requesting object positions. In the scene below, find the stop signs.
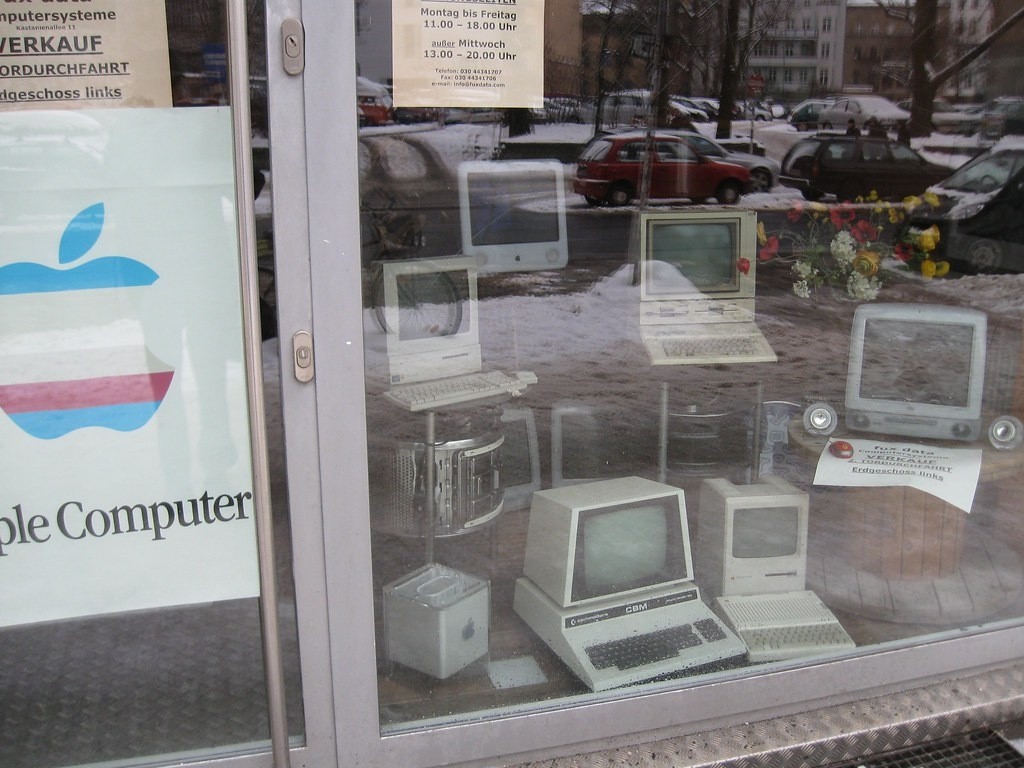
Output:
[747,74,764,94]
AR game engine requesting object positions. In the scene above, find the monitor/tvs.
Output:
[366,155,988,607]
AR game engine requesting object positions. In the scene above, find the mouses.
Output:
[828,441,854,458]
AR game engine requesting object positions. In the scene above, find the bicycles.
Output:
[256,196,461,341]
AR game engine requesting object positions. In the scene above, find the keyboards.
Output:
[384,369,526,412]
[661,338,760,358]
[740,622,854,652]
[585,617,728,671]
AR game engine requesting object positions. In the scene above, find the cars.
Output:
[896,98,977,137]
[360,133,476,237]
[448,89,551,123]
[786,100,836,131]
[573,133,758,208]
[183,42,395,126]
[586,90,773,126]
[980,96,1024,136]
[618,130,780,193]
[892,149,1024,277]
[958,105,984,114]
[542,97,589,124]
[818,97,910,131]
[391,89,450,124]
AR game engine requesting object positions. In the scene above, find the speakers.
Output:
[802,402,838,438]
[988,414,1024,452]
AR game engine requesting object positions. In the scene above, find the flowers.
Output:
[738,191,951,315]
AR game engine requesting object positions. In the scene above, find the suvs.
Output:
[778,131,958,203]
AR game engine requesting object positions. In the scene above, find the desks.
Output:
[786,410,1023,628]
[368,391,517,564]
[642,363,781,484]
